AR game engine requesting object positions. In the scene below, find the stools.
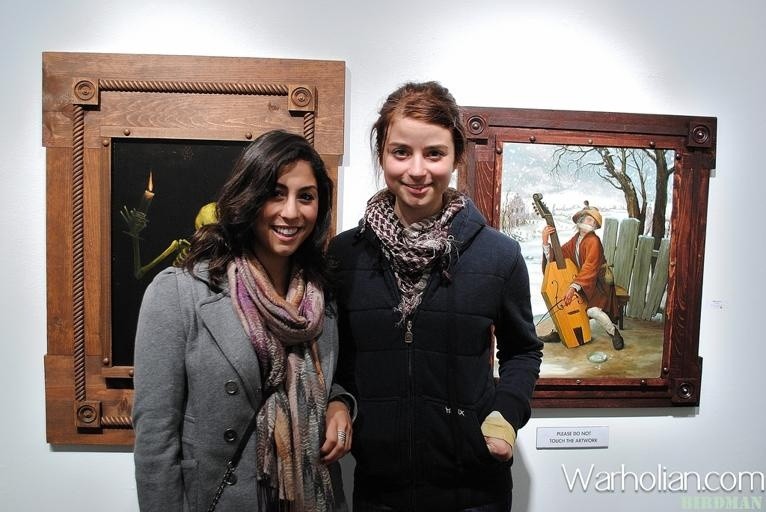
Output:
[614,283,629,331]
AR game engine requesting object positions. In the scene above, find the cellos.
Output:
[531,192,594,350]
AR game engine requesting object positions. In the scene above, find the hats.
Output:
[571,207,605,229]
[605,268,614,286]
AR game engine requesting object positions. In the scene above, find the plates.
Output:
[587,351,608,362]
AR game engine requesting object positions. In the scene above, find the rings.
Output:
[337,430,348,441]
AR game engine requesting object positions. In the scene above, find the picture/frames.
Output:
[42,52,346,451]
[457,107,717,407]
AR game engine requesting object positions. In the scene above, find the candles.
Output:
[137,169,156,215]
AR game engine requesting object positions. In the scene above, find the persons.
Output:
[318,79,544,511]
[131,127,360,511]
[537,204,625,351]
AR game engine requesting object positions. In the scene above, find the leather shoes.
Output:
[539,332,560,342]
[606,326,623,350]
